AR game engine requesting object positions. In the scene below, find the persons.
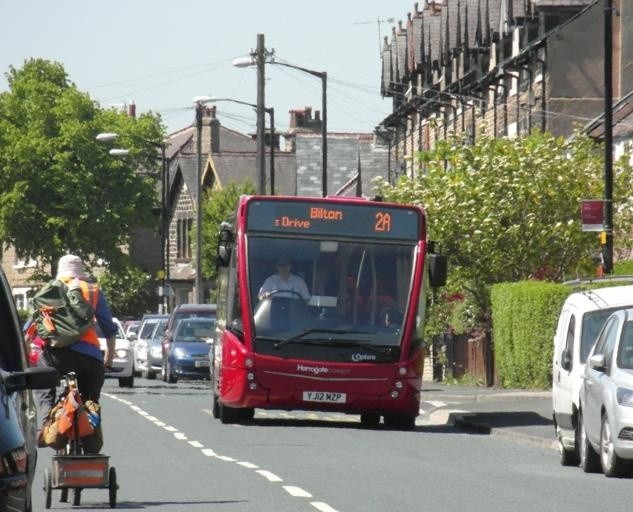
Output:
[256,257,310,306]
[22,254,117,454]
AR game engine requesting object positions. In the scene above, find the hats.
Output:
[56,254,90,279]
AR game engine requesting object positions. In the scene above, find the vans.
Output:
[550,284,633,466]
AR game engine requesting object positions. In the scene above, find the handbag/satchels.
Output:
[43,391,103,454]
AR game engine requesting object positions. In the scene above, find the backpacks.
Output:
[30,278,94,349]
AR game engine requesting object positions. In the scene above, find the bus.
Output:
[210,192,446,432]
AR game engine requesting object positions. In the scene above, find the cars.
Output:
[92,301,218,389]
[0,263,66,512]
[575,307,632,478]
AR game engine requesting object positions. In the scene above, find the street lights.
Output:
[233,56,328,201]
[106,148,171,314]
[93,131,171,314]
[191,94,274,194]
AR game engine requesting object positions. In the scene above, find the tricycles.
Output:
[31,349,120,510]
